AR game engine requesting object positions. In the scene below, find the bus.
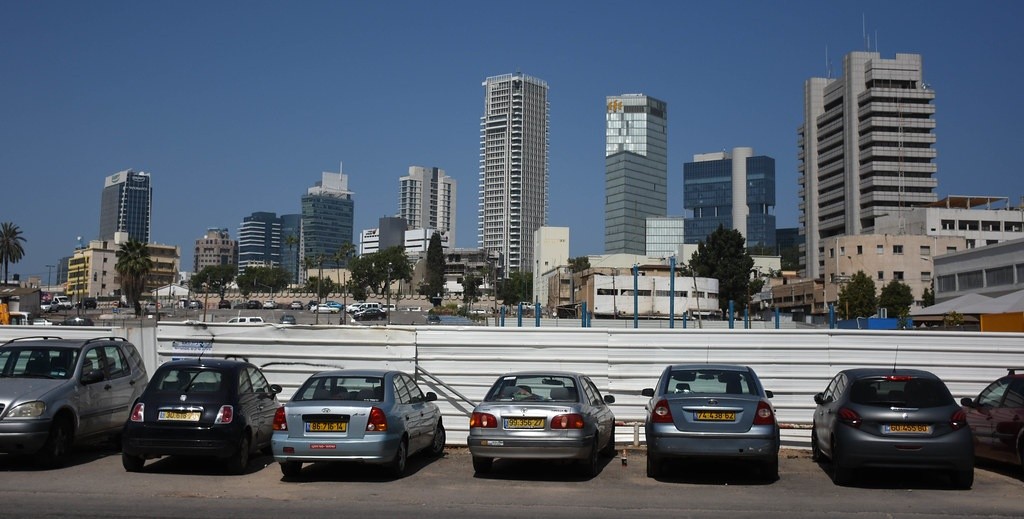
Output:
[10,312,33,325]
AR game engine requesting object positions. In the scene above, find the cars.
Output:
[811,368,974,490]
[467,371,616,476]
[40,301,59,313]
[353,308,387,321]
[308,300,345,314]
[641,364,781,479]
[280,314,296,324]
[189,299,204,309]
[960,367,1024,468]
[271,369,446,478]
[426,315,440,325]
[469,308,487,315]
[219,299,279,309]
[120,359,282,475]
[528,303,542,310]
[291,300,303,310]
[34,317,94,326]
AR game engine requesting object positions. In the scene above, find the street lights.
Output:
[46,265,55,291]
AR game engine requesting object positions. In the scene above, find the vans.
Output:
[346,301,397,313]
[227,317,264,324]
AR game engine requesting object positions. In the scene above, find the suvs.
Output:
[0,336,149,469]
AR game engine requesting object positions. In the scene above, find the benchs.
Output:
[161,382,216,392]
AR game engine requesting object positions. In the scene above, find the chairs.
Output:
[329,385,349,400]
[96,358,118,375]
[503,385,521,399]
[904,381,934,399]
[517,385,534,396]
[727,378,743,393]
[48,356,67,373]
[851,381,879,402]
[74,358,92,373]
[25,359,49,375]
[673,383,691,393]
[888,389,903,401]
[357,389,376,401]
[550,388,569,401]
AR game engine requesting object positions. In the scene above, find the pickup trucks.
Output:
[513,302,532,310]
[53,296,97,309]
